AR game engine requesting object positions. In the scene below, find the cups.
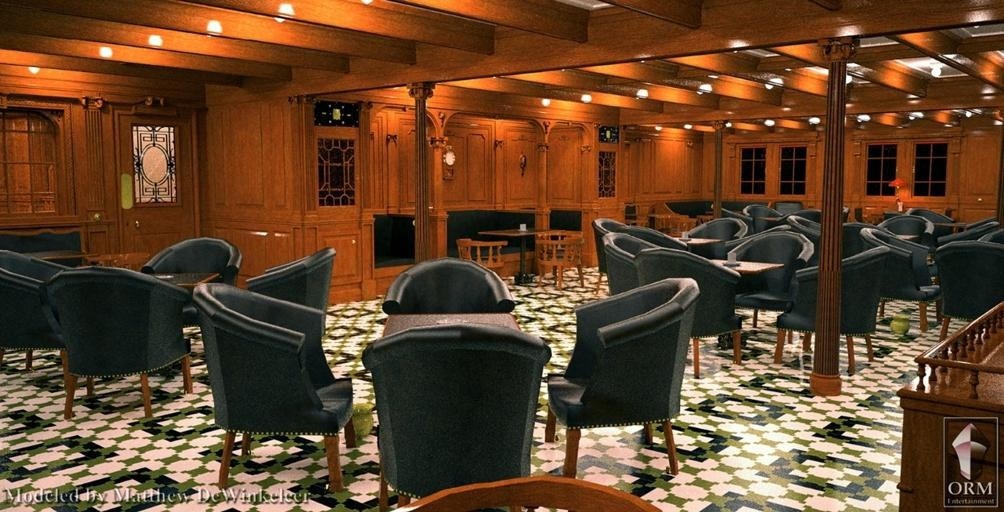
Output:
[680,230,688,239]
[897,201,904,212]
[727,252,737,264]
[520,224,526,232]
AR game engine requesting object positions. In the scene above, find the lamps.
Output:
[441,145,458,180]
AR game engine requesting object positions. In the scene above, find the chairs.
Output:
[243,245,337,338]
[138,236,240,284]
[189,280,360,493]
[455,198,1004,477]
[383,257,514,313]
[39,265,191,419]
[0,246,71,364]
[385,475,660,512]
[361,325,551,511]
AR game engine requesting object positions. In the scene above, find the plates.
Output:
[678,238,692,242]
[722,262,740,267]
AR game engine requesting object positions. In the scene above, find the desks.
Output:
[148,272,220,290]
[380,312,518,342]
